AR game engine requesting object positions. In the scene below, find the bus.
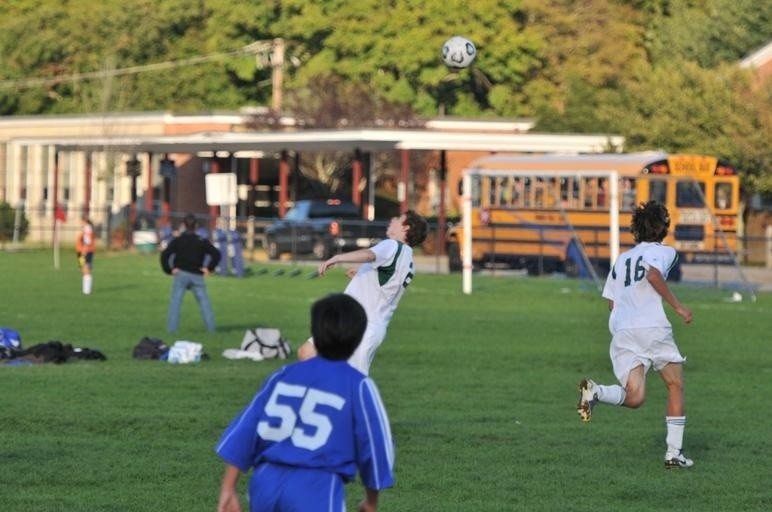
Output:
[443,151,740,282]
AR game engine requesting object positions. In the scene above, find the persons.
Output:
[295,207,430,381]
[157,211,224,334]
[576,201,697,469]
[212,290,400,512]
[72,213,99,299]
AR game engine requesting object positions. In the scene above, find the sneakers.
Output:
[663,452,694,470]
[576,377,600,424]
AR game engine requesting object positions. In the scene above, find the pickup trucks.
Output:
[262,198,390,261]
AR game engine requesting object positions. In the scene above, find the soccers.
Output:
[442,35,477,69]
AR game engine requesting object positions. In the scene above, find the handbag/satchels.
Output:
[0,327,23,351]
[242,324,290,362]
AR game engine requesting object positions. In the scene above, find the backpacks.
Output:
[130,335,168,361]
[166,338,204,366]
